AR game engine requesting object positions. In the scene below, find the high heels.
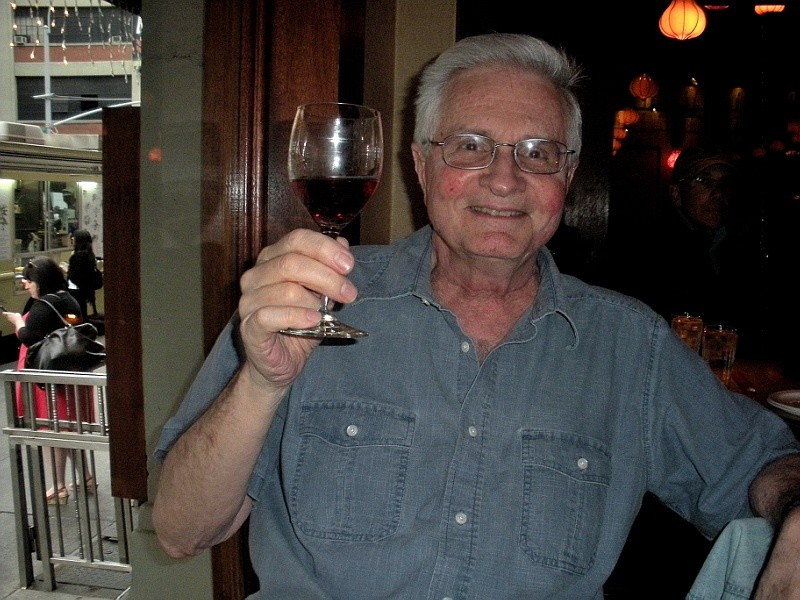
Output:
[46,485,69,505]
[69,476,98,492]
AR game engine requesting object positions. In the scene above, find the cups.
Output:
[671,312,703,354]
[703,325,738,389]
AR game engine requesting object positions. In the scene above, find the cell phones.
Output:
[0,305,7,312]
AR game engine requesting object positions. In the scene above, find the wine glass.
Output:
[279,103,383,338]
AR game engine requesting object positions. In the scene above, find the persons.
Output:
[3,256,98,504]
[150,30,800,599]
[59,228,99,319]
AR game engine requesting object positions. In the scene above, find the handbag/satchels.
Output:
[94,267,104,291]
[25,299,107,391]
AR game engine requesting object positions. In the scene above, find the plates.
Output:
[766,390,800,417]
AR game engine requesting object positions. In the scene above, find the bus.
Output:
[0,121,103,339]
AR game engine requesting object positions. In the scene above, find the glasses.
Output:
[27,258,43,271]
[426,134,578,176]
